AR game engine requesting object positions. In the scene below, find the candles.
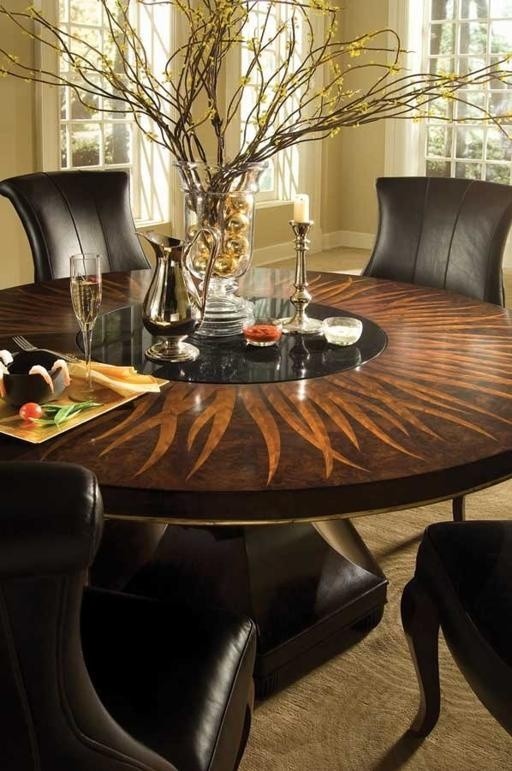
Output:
[293,193,309,223]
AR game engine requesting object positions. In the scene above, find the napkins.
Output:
[65,360,161,398]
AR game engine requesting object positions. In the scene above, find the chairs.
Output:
[359,176,511,521]
[2,168,153,284]
[2,460,261,770]
[398,520,509,739]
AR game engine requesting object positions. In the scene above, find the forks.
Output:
[13,333,78,363]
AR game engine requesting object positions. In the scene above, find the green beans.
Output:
[28,399,111,425]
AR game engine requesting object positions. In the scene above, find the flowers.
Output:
[0,0,512,281]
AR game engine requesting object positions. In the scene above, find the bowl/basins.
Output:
[1,351,72,405]
[243,317,282,348]
[322,316,364,345]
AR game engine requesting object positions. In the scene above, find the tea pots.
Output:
[132,221,221,364]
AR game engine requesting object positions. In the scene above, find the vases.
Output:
[180,184,254,337]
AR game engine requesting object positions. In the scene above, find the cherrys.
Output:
[19,403,43,420]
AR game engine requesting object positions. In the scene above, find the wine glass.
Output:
[68,254,112,400]
